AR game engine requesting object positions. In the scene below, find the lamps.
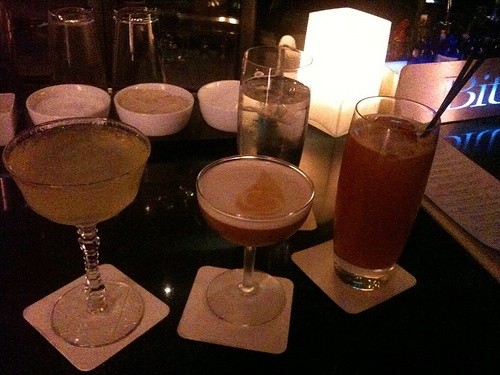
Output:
[296,7,392,138]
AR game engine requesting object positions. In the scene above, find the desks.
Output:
[0,57,500,375]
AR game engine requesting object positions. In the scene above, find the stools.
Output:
[112,6,166,89]
[47,4,108,95]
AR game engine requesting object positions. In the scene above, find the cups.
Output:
[40,5,105,90]
[236,45,311,168]
[109,6,173,90]
[332,96,441,292]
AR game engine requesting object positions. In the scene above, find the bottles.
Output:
[407,0,500,64]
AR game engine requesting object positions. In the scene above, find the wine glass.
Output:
[195,155,316,327]
[2,116,152,348]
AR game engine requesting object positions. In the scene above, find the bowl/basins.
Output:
[113,82,194,137]
[197,79,240,133]
[26,83,112,126]
